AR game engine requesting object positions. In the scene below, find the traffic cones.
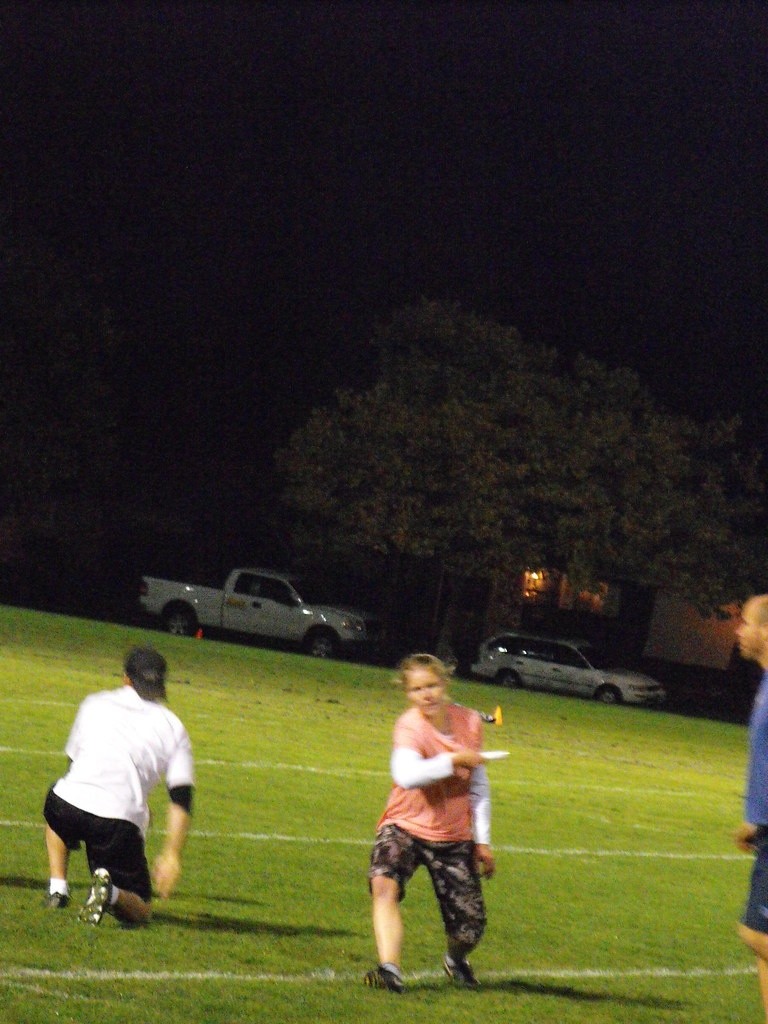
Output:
[194,627,204,640]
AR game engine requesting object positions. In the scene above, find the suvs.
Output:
[466,627,669,712]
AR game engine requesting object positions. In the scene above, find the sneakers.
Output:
[362,966,404,993]
[441,957,482,992]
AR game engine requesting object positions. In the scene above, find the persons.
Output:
[362,654,495,995]
[43,648,195,927]
[735,594,768,1024]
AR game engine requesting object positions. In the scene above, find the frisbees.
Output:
[479,751,511,760]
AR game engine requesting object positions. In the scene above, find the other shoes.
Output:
[41,892,70,908]
[75,867,113,927]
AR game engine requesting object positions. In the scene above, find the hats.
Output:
[125,649,170,703]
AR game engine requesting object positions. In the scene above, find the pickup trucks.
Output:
[135,564,370,665]
